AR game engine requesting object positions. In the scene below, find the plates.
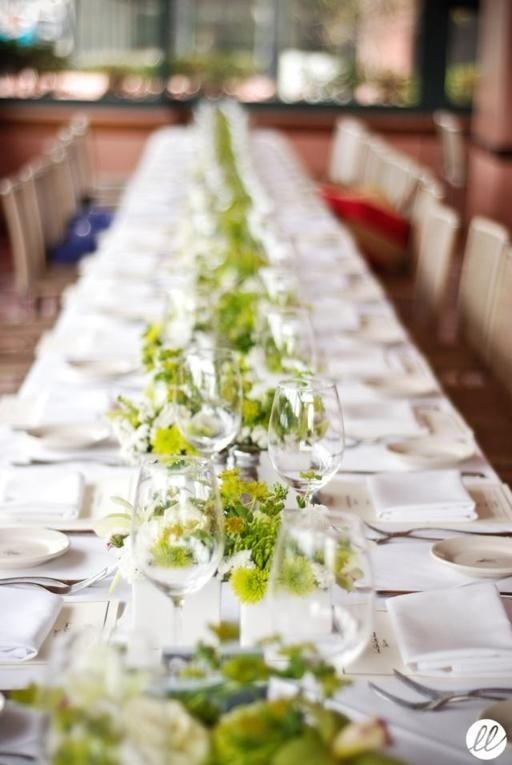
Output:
[2,100,512,763]
